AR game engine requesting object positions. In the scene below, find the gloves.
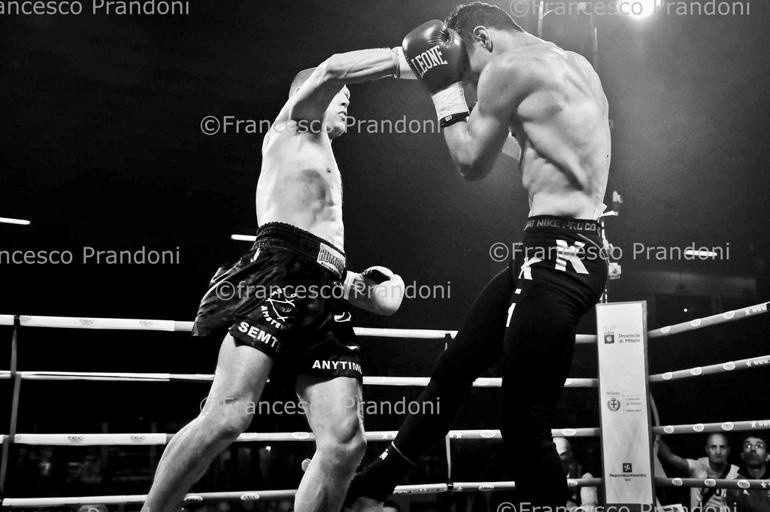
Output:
[392,19,471,94]
[343,265,405,316]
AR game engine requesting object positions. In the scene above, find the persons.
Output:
[137,47,417,512]
[301,1,611,512]
